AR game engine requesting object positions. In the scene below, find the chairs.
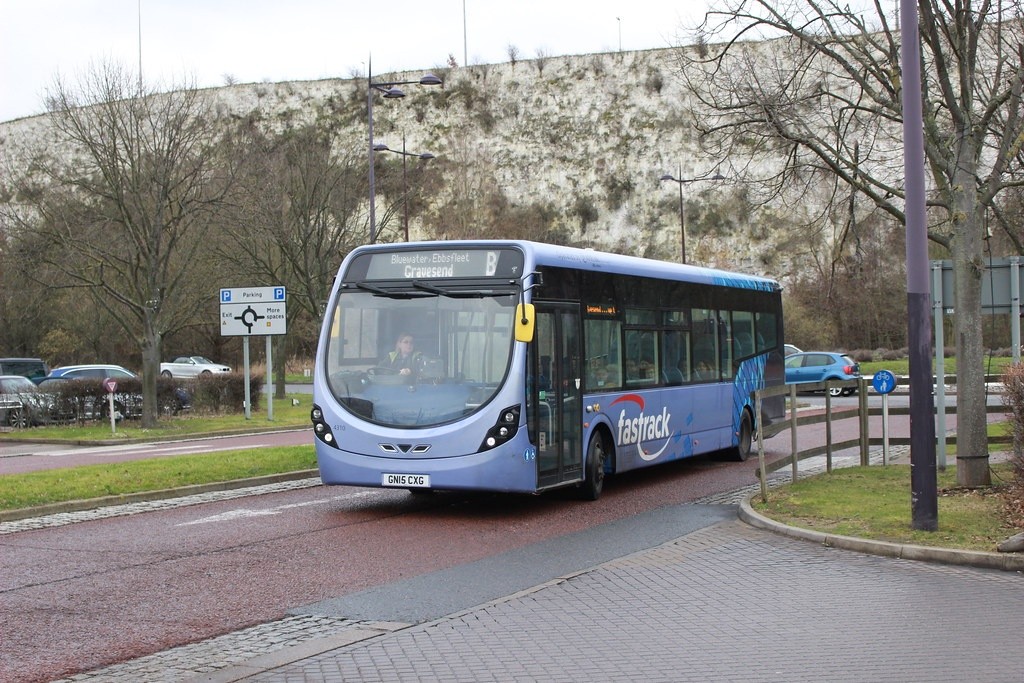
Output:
[542,319,761,391]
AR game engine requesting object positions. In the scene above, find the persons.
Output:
[366,331,422,380]
[593,356,713,389]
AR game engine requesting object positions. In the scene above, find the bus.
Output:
[310,237,789,503]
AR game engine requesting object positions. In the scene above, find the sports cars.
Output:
[159,355,233,379]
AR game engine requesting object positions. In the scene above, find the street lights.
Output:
[366,73,442,245]
[371,130,438,244]
[658,162,727,265]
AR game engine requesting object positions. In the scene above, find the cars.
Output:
[0,358,192,431]
[783,343,804,356]
[783,351,862,398]
